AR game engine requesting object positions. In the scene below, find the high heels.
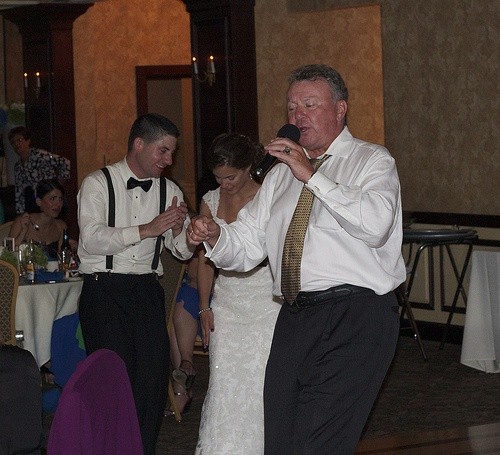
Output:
[163,388,194,418]
[174,360,197,390]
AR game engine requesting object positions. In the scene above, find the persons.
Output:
[7,126,71,217]
[185,63,407,455]
[162,180,218,415]
[7,178,78,261]
[195,133,285,455]
[76,113,194,455]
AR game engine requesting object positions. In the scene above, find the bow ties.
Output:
[126,176,153,192]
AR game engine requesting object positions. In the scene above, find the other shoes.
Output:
[193,337,210,355]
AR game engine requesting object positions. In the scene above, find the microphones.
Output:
[250,123,301,183]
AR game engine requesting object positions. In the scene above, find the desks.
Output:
[14,261,86,384]
[394,228,478,360]
[461,250,500,374]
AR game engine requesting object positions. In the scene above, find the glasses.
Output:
[18,137,26,142]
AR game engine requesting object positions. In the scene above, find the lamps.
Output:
[192,54,216,86]
[24,71,44,100]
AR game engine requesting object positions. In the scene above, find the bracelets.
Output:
[198,307,212,315]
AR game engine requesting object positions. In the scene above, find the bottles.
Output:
[25,239,39,284]
[61,229,71,250]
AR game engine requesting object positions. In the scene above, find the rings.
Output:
[189,230,194,237]
[282,146,291,154]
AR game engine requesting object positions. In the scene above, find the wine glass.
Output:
[57,251,73,282]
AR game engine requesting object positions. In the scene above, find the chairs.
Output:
[0,219,189,455]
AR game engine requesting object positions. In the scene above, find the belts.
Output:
[281,284,368,310]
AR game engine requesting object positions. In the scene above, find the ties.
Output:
[281,154,333,308]
